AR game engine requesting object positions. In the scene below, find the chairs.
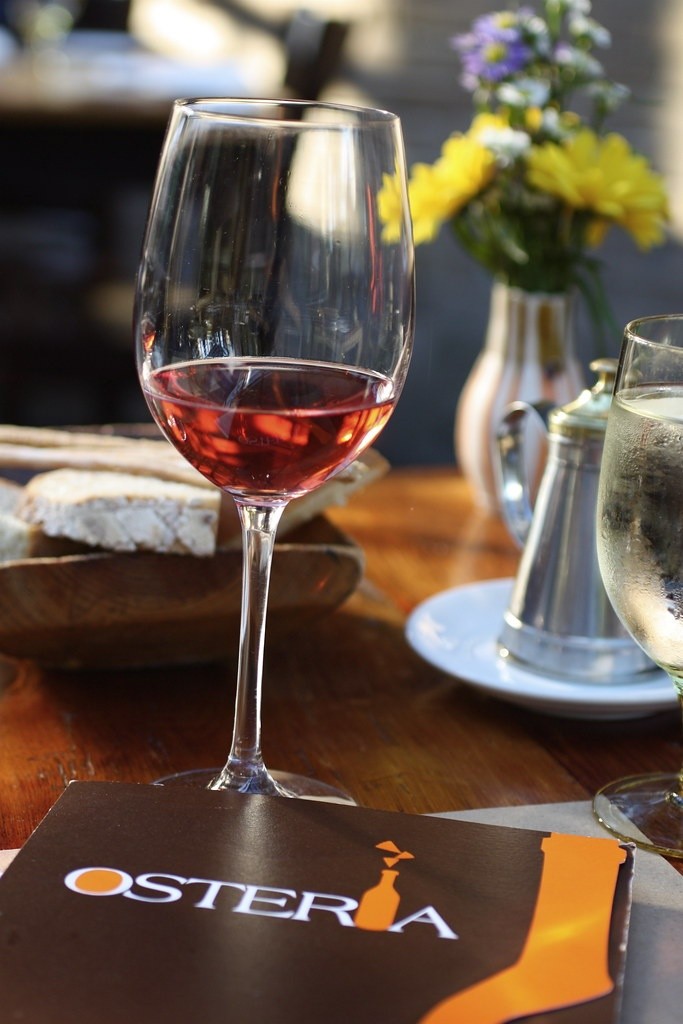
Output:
[0,0,348,411]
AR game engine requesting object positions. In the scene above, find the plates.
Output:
[406,574,677,723]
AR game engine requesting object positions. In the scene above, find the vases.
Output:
[454,285,590,517]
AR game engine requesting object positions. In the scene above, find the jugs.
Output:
[488,360,663,680]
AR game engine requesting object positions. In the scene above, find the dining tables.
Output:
[0,423,683,1024]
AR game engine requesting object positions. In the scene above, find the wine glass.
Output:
[594,316,682,861]
[135,100,418,818]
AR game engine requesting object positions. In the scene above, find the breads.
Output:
[0,425,389,566]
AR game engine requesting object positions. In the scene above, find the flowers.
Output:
[377,0,680,300]
